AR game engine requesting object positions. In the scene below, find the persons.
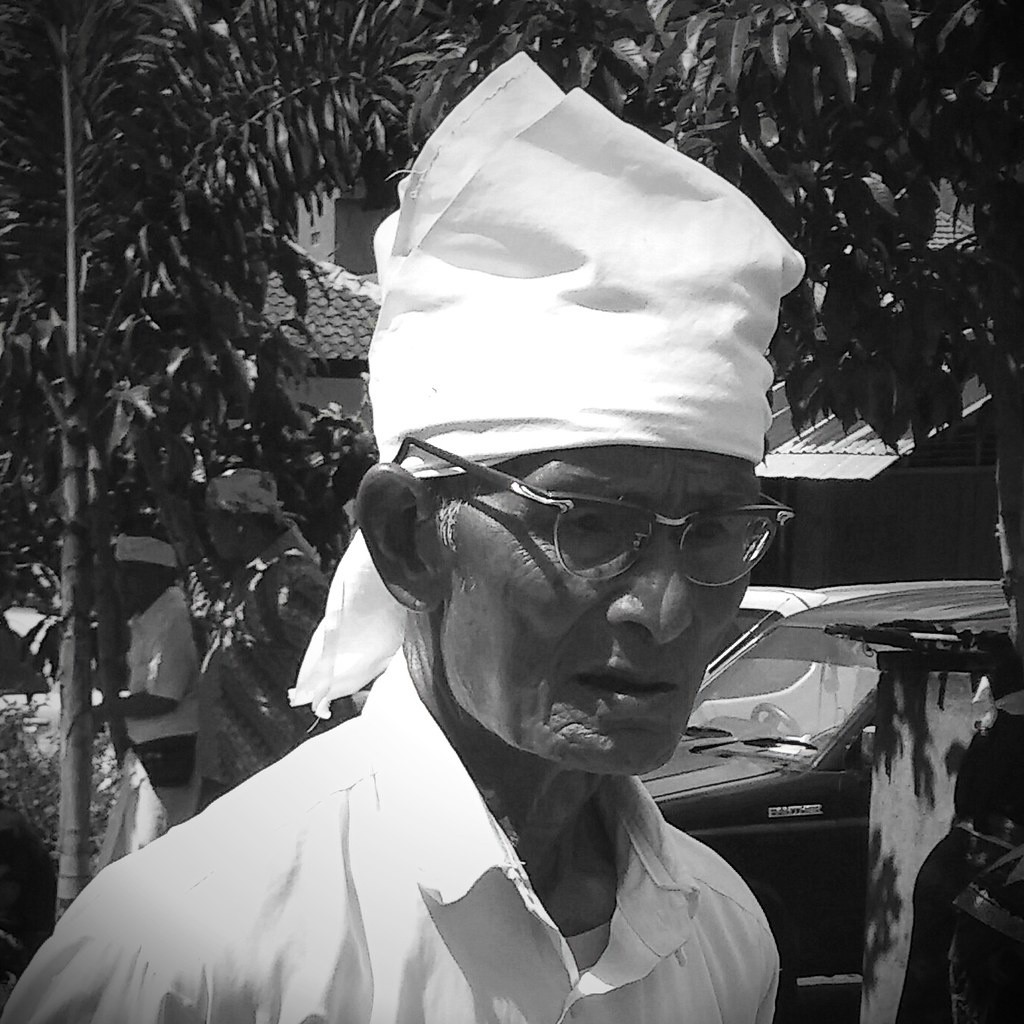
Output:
[201,456,358,817]
[87,534,201,876]
[0,49,805,1024]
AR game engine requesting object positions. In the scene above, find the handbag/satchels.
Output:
[132,733,198,787]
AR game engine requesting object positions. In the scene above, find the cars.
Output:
[685,576,1005,740]
[640,582,1024,997]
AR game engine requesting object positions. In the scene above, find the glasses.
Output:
[393,437,795,587]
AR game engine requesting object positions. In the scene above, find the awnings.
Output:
[753,375,990,479]
